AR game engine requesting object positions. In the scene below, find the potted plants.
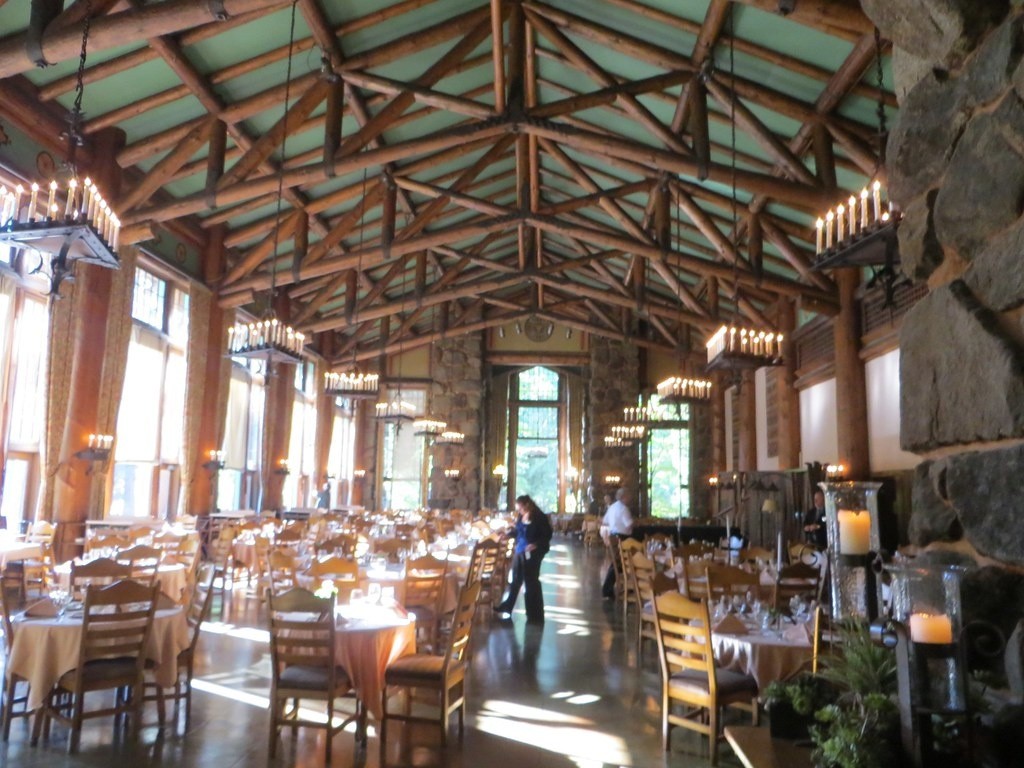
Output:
[754,613,1024,768]
[209,450,228,466]
[353,469,366,481]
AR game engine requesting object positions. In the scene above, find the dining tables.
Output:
[651,544,876,768]
[233,509,509,646]
[270,602,418,750]
[56,546,189,609]
[0,535,44,607]
[4,587,190,748]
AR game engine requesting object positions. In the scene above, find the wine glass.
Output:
[747,591,758,620]
[350,582,394,608]
[719,594,732,618]
[756,602,769,631]
[733,594,746,618]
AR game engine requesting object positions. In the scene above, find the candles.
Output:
[444,469,461,480]
[375,401,417,420]
[0,176,124,262]
[705,324,785,365]
[323,371,380,393]
[908,609,953,645]
[826,464,844,477]
[411,421,466,445]
[623,407,664,425]
[814,180,905,259]
[278,458,290,471]
[708,477,718,486]
[225,318,306,359]
[657,376,713,401]
[88,433,114,452]
[605,475,620,488]
[603,426,646,449]
[835,500,871,557]
[492,465,508,479]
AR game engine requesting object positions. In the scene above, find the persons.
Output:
[314,483,330,512]
[599,484,635,602]
[804,493,829,552]
[493,494,553,625]
[601,492,613,517]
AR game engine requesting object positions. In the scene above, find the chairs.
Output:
[0,501,890,768]
